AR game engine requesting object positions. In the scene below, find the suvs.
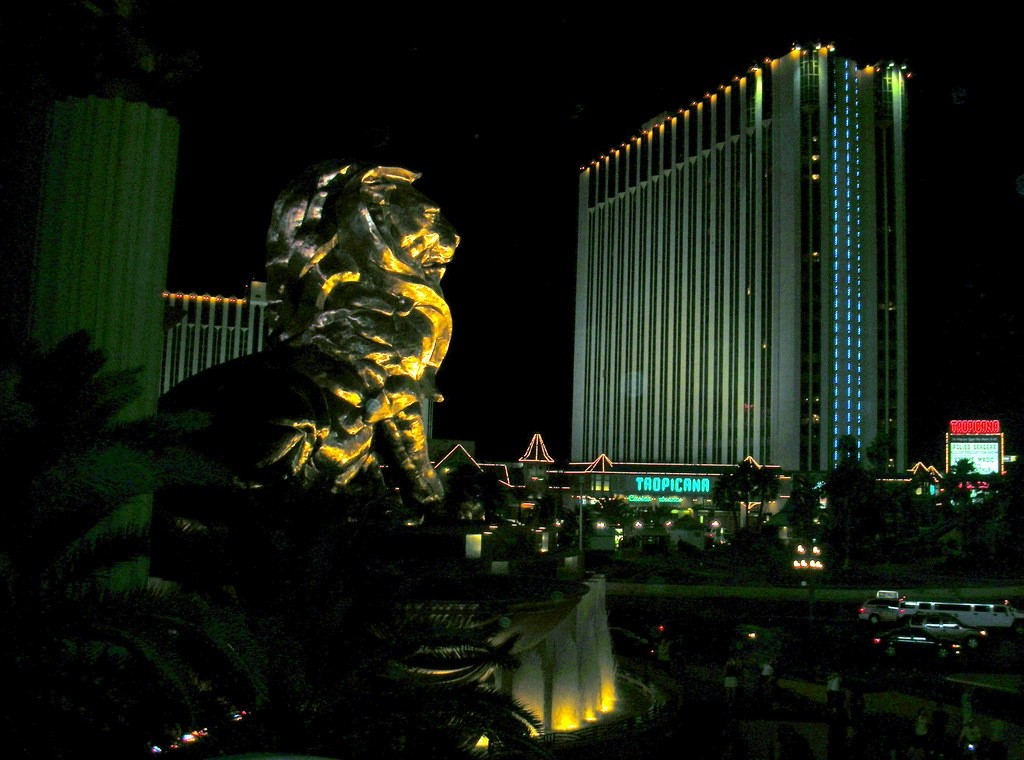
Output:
[898,601,1024,633]
[906,613,990,649]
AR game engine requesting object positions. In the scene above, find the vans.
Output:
[640,625,779,708]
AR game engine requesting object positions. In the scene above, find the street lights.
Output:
[792,542,825,622]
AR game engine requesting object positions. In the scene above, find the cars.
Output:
[859,598,901,623]
[874,627,963,658]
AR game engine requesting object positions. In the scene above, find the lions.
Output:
[155,162,461,518]
